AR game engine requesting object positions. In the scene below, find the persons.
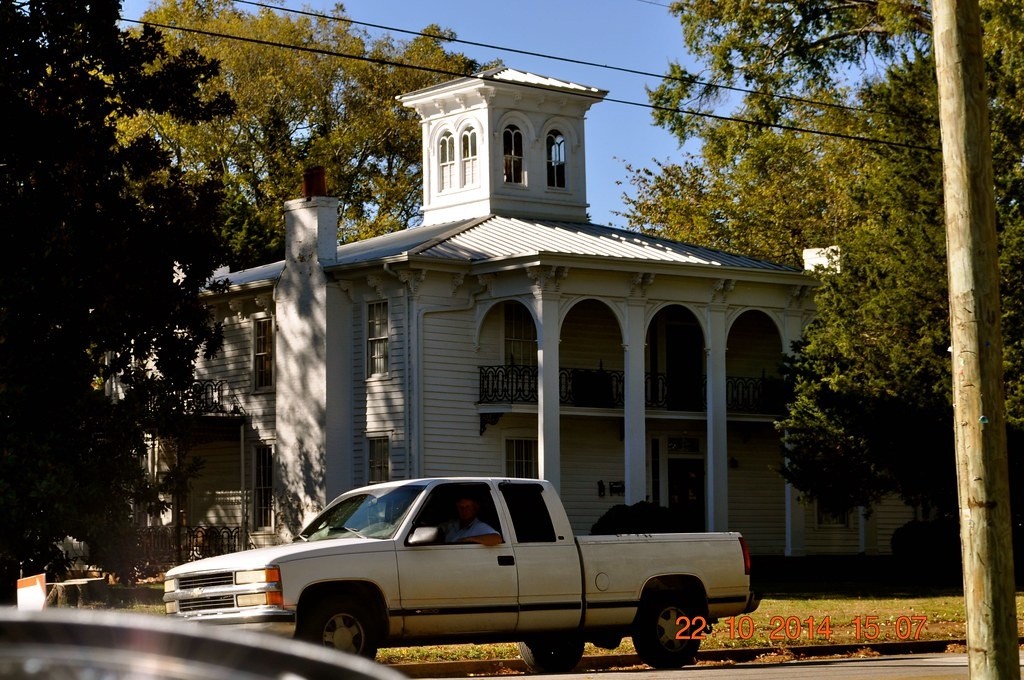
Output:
[445,495,502,547]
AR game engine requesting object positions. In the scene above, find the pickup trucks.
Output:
[163,477,760,674]
